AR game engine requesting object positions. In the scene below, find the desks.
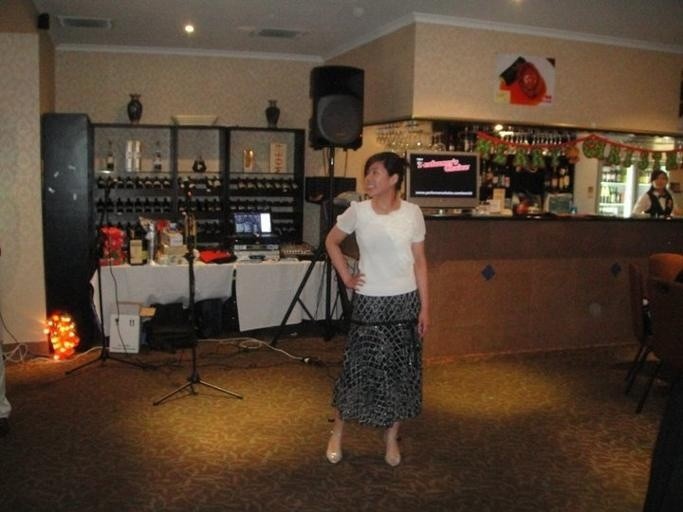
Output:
[95,254,347,339]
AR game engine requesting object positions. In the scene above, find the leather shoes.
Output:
[326,430,342,464]
[382,427,401,467]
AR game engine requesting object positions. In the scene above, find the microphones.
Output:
[186,189,194,216]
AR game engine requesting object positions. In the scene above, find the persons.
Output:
[633,169,682,218]
[326,152,430,468]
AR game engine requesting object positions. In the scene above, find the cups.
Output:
[487,199,501,213]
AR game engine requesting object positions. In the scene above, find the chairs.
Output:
[623,250,682,415]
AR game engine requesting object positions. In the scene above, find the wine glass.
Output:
[374,121,573,158]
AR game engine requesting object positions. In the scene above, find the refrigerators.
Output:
[573,132,638,219]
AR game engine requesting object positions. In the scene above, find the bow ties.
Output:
[657,193,667,200]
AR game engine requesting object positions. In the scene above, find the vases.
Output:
[265,99,281,126]
[127,93,143,124]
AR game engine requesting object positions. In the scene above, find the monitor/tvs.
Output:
[405,148,480,208]
[231,210,274,235]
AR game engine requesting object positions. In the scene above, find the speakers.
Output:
[308,64,365,150]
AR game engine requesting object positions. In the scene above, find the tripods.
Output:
[269,161,352,348]
[151,255,245,407]
[64,197,151,384]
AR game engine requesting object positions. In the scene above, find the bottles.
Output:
[549,165,572,190]
[480,159,512,189]
[94,172,299,244]
[152,141,162,172]
[106,141,114,172]
[191,156,206,173]
[599,162,626,218]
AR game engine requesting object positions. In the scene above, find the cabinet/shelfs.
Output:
[91,122,306,249]
